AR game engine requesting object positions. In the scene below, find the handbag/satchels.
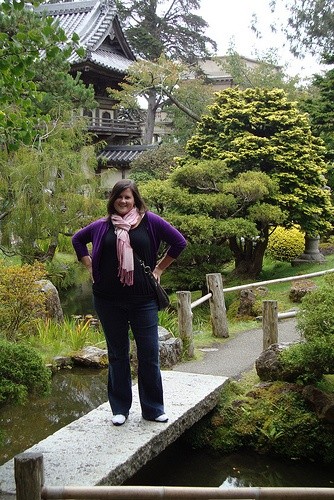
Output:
[144,266,170,310]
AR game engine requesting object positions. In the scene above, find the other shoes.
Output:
[154,414,169,422]
[111,414,126,425]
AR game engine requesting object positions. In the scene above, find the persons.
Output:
[71,178,188,425]
